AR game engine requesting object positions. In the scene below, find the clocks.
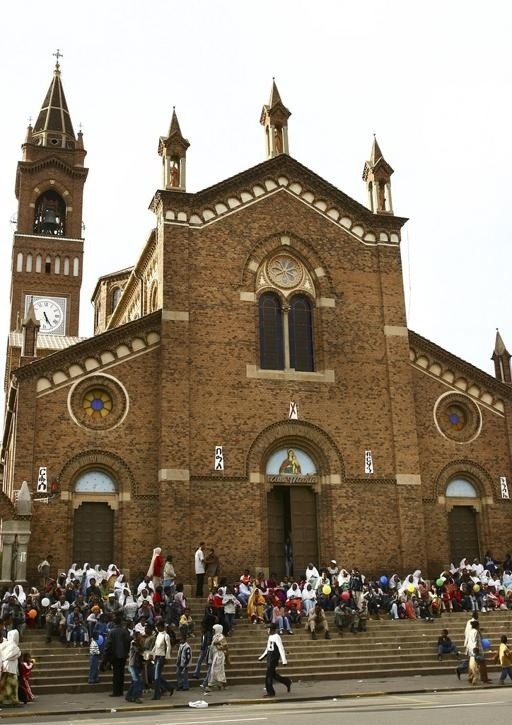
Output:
[21,290,70,337]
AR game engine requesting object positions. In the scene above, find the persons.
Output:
[291,456,299,474]
[279,448,301,475]
[2,542,511,708]
